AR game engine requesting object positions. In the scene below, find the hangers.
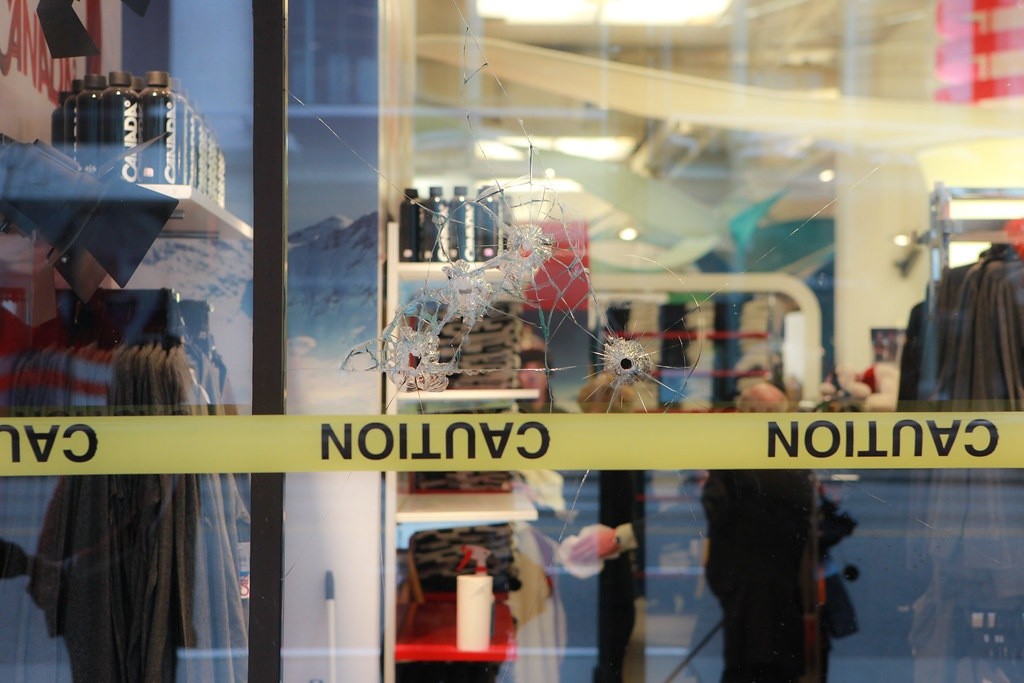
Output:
[37,286,216,351]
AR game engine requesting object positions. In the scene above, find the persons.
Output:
[513,349,577,414]
[561,370,647,683]
[699,380,836,683]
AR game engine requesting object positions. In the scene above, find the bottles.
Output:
[51,72,226,211]
[400,185,512,262]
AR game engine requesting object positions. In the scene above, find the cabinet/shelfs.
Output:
[382,223,540,683]
